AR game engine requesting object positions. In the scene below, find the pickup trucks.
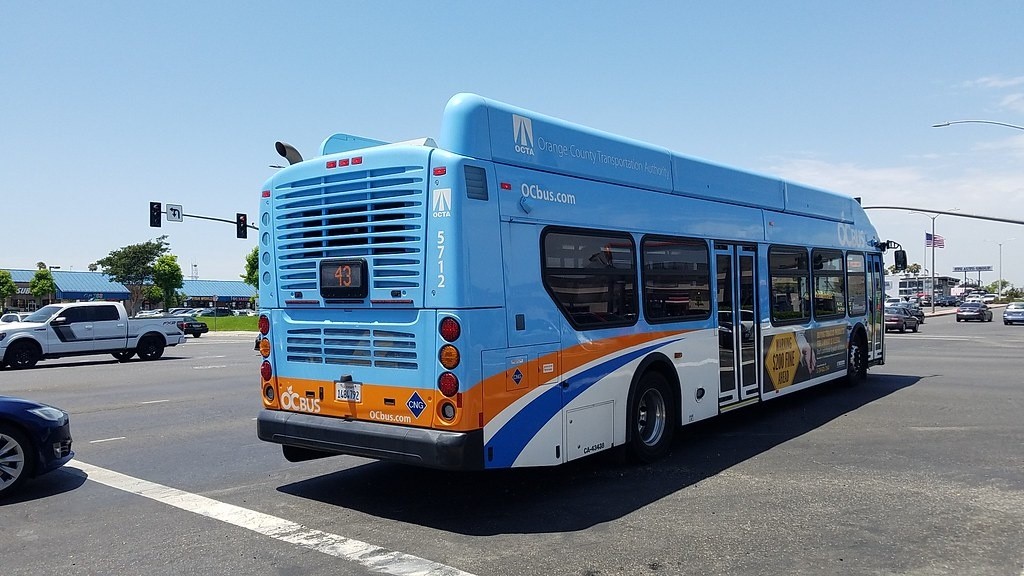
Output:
[0,300,187,371]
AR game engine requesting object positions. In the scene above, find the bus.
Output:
[254,92,907,484]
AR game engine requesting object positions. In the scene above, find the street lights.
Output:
[983,237,1017,301]
[907,207,960,314]
[48,266,61,305]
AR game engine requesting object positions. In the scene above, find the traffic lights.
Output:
[149,201,161,227]
[236,213,247,239]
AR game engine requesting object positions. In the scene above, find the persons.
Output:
[795,330,816,372]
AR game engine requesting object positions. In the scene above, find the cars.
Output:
[179,316,209,338]
[0,311,34,323]
[129,306,259,318]
[884,290,999,324]
[956,301,993,322]
[0,395,75,502]
[1002,302,1024,325]
[718,309,754,346]
[884,307,921,334]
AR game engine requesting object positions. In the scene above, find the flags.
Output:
[926,233,945,248]
